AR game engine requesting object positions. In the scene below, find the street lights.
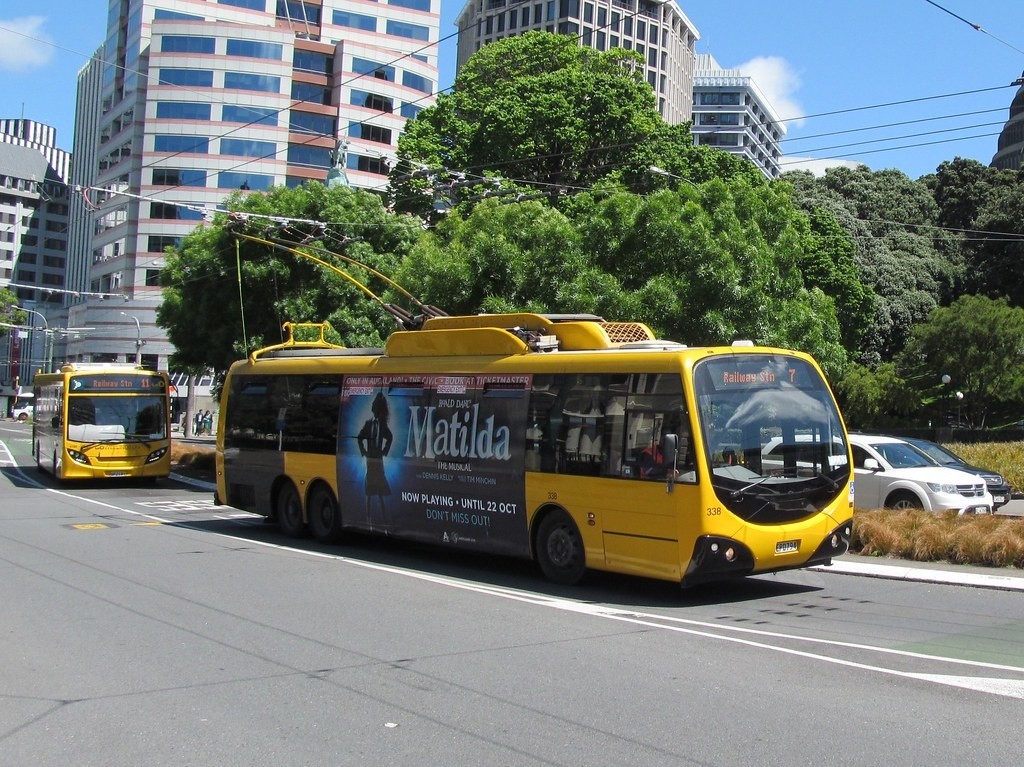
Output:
[954,389,962,426]
[10,305,48,373]
[120,311,142,365]
[942,372,951,430]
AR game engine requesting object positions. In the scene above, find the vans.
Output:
[13,394,36,420]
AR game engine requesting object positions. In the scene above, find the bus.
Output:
[33,362,179,482]
[213,314,854,593]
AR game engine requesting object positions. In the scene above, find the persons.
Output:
[195,409,204,436]
[202,410,212,436]
[357,393,394,529]
[638,429,679,482]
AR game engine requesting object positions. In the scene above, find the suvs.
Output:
[759,434,1011,524]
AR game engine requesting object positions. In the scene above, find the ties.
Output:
[372,420,378,449]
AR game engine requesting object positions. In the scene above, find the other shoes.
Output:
[195,433,196,436]
[198,434,199,436]
